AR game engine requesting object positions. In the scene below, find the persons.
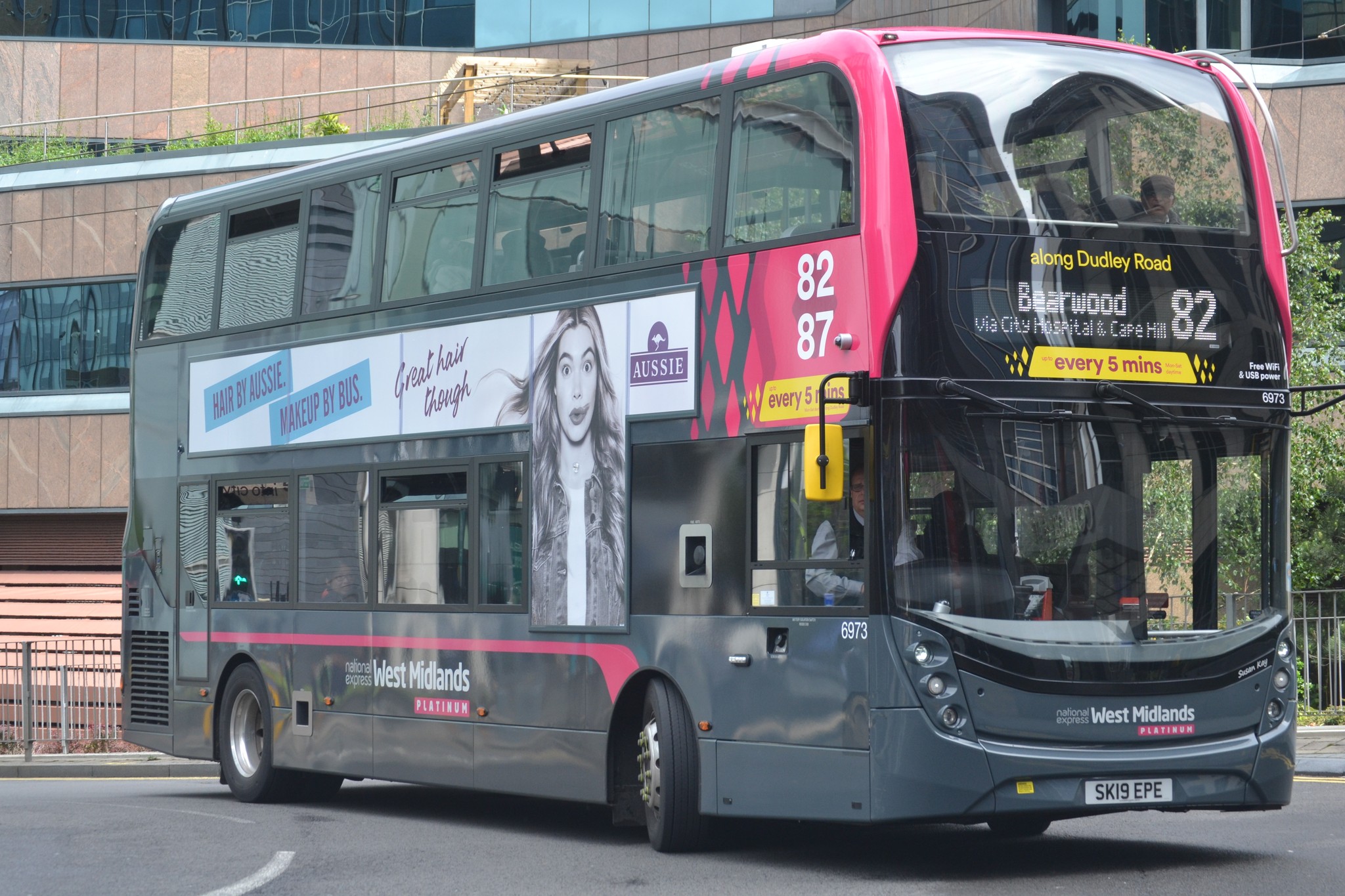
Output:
[479,303,626,626]
[322,558,360,602]
[1133,174,1187,226]
[805,461,926,604]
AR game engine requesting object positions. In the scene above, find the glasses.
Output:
[851,484,865,492]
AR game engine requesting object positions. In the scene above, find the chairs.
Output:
[1018,194,1142,221]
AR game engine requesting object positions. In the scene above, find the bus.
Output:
[120,26,1345,857]
